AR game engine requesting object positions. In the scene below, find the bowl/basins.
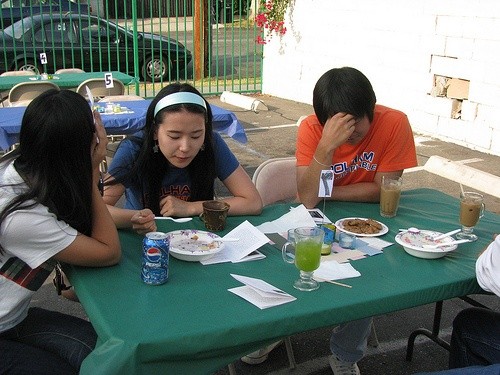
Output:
[395,229,459,259]
[166,229,225,262]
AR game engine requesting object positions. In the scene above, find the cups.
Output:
[316,225,336,256]
[339,232,356,249]
[199,200,230,231]
[288,227,296,254]
[83,94,121,113]
[379,173,403,219]
[36,73,53,80]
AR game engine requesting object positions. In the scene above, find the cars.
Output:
[0,14,192,83]
[0,0,92,31]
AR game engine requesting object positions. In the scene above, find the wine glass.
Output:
[456,191,485,242]
[282,226,325,292]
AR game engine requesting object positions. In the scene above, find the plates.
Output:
[334,217,389,236]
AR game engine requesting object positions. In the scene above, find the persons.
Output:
[102,83,262,237]
[0,89,121,375]
[241,67,418,375]
[448,234,500,370]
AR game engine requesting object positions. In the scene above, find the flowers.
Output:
[255,0,289,44]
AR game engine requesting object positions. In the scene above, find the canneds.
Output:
[141,232,169,286]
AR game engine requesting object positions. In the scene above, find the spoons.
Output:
[422,239,471,249]
[154,216,193,223]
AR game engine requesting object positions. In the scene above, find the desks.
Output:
[0,71,135,93]
[0,100,247,160]
[60,188,500,375]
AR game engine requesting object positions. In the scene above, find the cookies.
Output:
[342,218,383,235]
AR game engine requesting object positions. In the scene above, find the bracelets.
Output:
[313,155,332,167]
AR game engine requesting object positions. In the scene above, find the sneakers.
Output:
[241,340,282,365]
[328,353,360,375]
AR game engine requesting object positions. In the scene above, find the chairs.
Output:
[0,68,145,173]
[228,156,379,375]
